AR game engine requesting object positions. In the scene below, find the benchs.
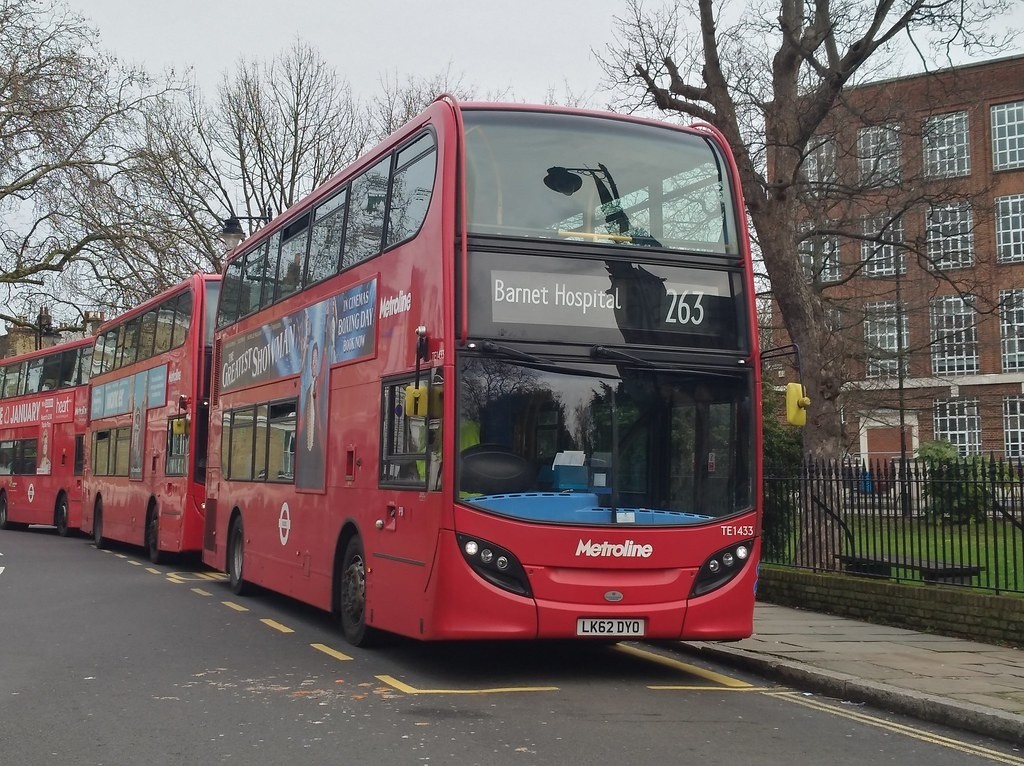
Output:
[834,552,986,586]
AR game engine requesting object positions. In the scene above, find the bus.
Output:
[0,331,96,537]
[77,270,223,565]
[203,97,813,649]
